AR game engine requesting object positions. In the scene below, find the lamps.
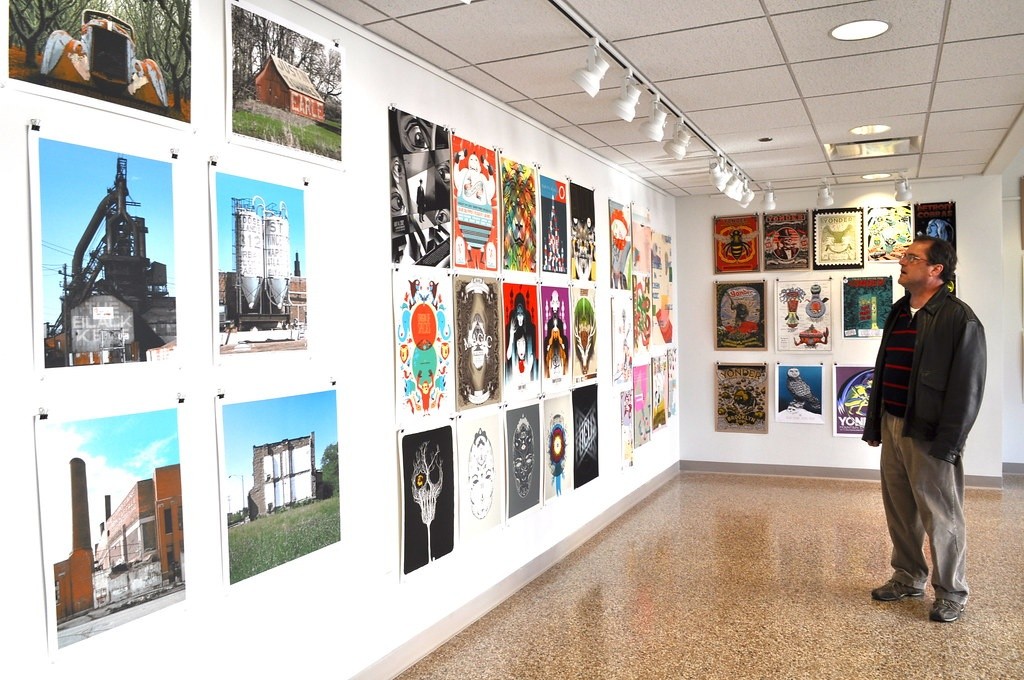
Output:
[664,117,691,161]
[608,67,642,124]
[893,171,913,201]
[759,182,776,211]
[568,38,608,98]
[638,93,667,142]
[707,151,754,209]
[816,178,835,206]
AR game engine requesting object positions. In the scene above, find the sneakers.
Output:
[929,598,965,622]
[872,579,925,600]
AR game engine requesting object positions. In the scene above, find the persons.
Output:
[862,236,987,621]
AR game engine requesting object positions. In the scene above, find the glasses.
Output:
[900,253,934,265]
